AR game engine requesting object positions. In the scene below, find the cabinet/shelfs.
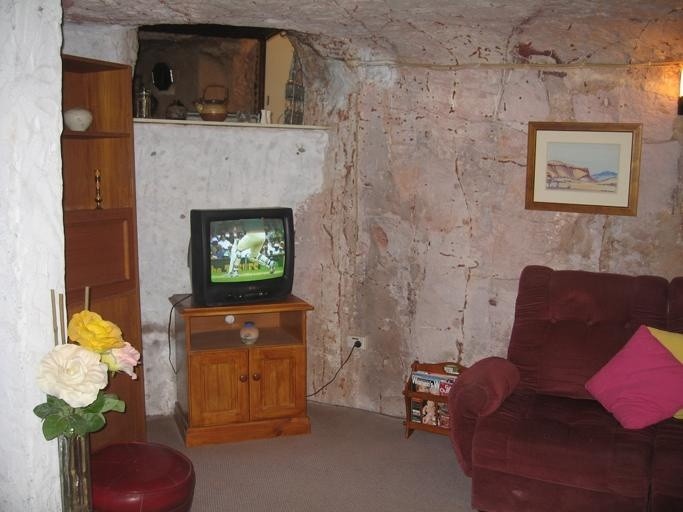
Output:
[169,293,314,448]
[62,54,146,440]
[402,359,469,439]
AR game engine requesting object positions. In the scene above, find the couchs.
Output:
[447,265,683,511]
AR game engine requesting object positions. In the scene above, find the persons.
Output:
[211,218,284,278]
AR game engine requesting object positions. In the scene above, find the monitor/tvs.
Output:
[191,207,294,307]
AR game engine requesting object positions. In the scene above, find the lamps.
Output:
[678,68,683,116]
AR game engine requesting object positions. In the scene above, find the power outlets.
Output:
[345,334,368,351]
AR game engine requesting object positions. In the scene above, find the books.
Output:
[411,371,460,430]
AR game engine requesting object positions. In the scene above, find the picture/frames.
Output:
[524,120,642,218]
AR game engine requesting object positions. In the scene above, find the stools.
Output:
[91,442,196,512]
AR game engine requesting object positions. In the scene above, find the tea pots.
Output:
[191,84,229,122]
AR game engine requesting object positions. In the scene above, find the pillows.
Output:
[583,324,683,431]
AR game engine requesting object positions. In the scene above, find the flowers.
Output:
[33,309,141,444]
